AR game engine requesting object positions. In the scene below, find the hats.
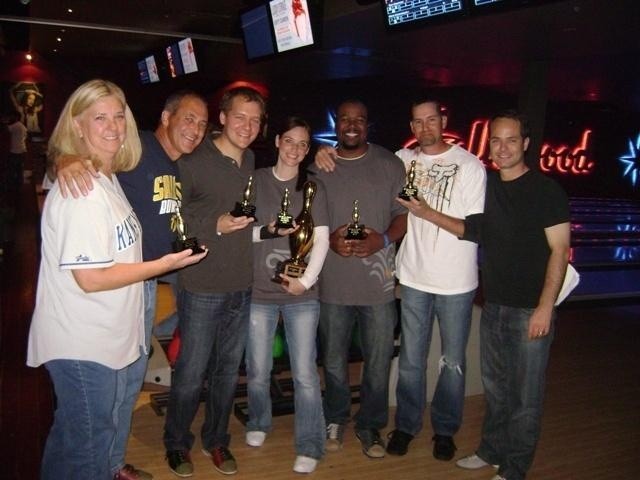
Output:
[211,78,272,101]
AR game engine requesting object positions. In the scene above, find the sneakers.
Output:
[454,452,503,470]
[113,462,155,480]
[164,443,196,478]
[244,430,269,448]
[324,422,347,454]
[430,432,459,463]
[200,445,239,476]
[386,427,416,458]
[354,427,388,459]
[291,453,320,474]
